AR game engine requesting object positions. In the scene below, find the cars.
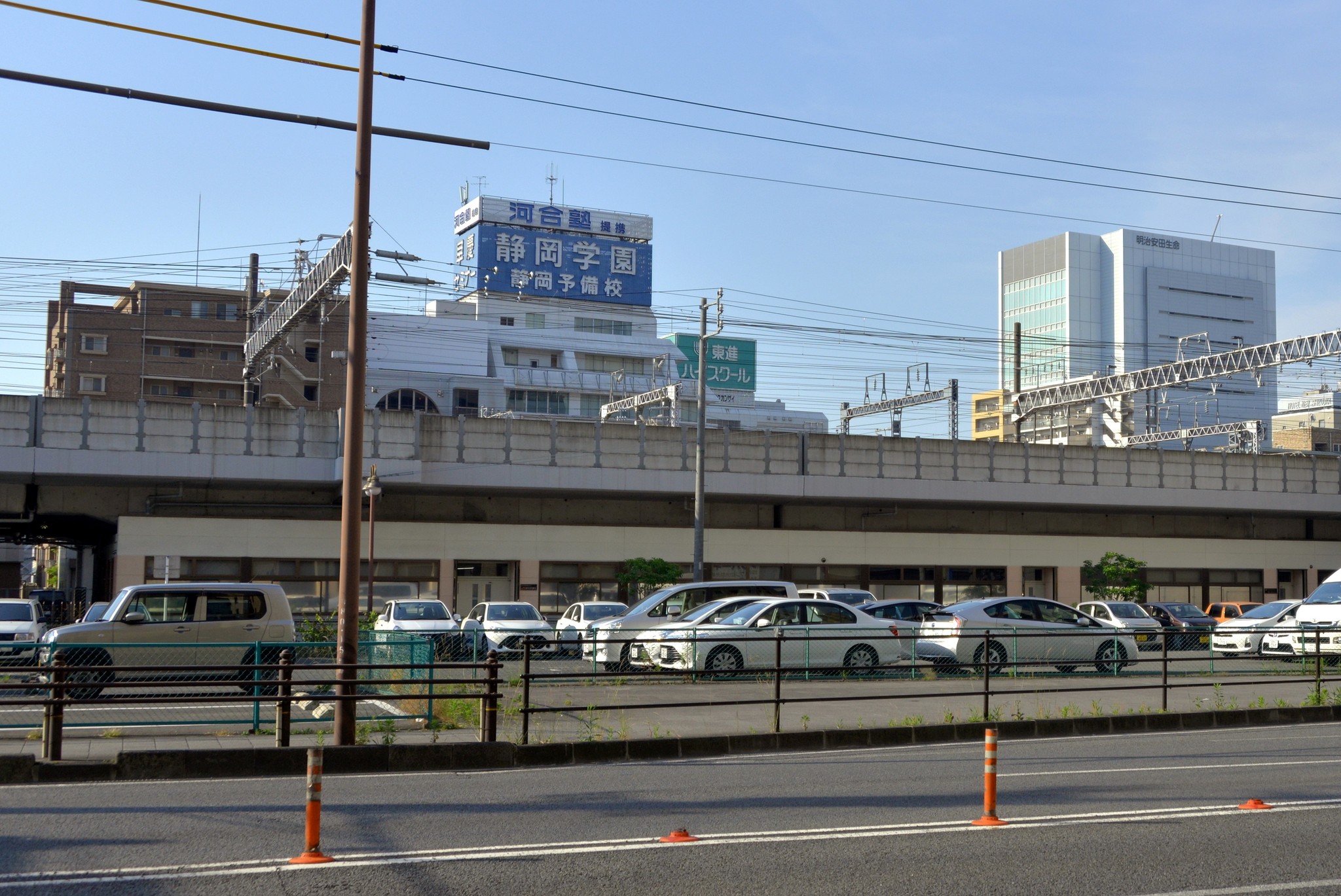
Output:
[1137,602,1220,649]
[914,595,1139,673]
[1075,600,1164,651]
[658,598,902,678]
[628,595,824,679]
[460,601,555,660]
[373,598,461,659]
[540,591,570,616]
[853,599,945,659]
[1205,601,1264,625]
[556,601,629,659]
[1212,599,1305,659]
[74,601,158,625]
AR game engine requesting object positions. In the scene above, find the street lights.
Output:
[362,463,382,623]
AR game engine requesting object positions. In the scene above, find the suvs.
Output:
[39,582,296,700]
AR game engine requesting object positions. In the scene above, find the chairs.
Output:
[1097,610,1104,618]
[397,607,408,621]
[685,593,698,614]
[489,609,497,620]
[1020,604,1033,620]
[423,608,436,620]
[901,606,914,622]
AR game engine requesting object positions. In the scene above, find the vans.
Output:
[582,582,801,673]
[28,589,68,625]
[797,588,878,611]
[1290,567,1341,667]
[0,598,47,667]
